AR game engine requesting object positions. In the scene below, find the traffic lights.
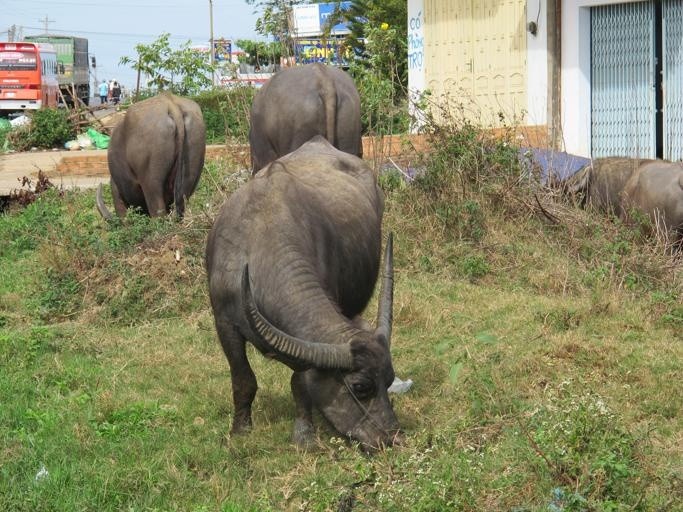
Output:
[92,57,96,68]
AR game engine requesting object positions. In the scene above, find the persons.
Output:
[98,80,108,104]
[109,79,119,101]
[120,85,127,96]
[110,81,121,106]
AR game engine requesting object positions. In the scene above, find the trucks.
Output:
[23,35,90,108]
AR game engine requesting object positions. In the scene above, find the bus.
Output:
[0,41,64,118]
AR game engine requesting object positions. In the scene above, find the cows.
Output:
[249,62,362,176]
[563,156,683,253]
[96,91,206,228]
[205,135,406,459]
